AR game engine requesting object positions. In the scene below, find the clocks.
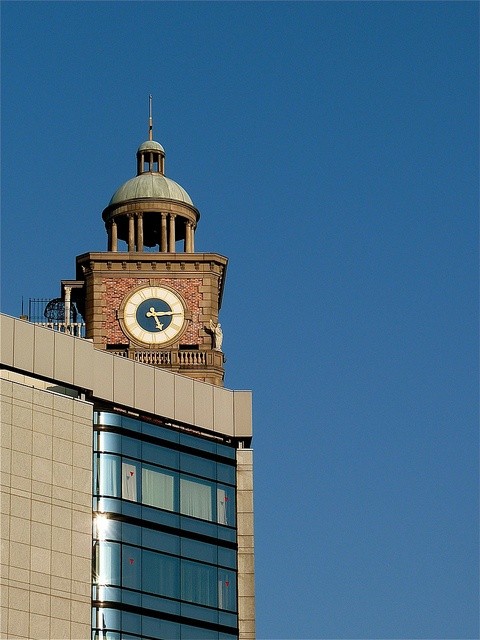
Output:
[115,278,193,348]
